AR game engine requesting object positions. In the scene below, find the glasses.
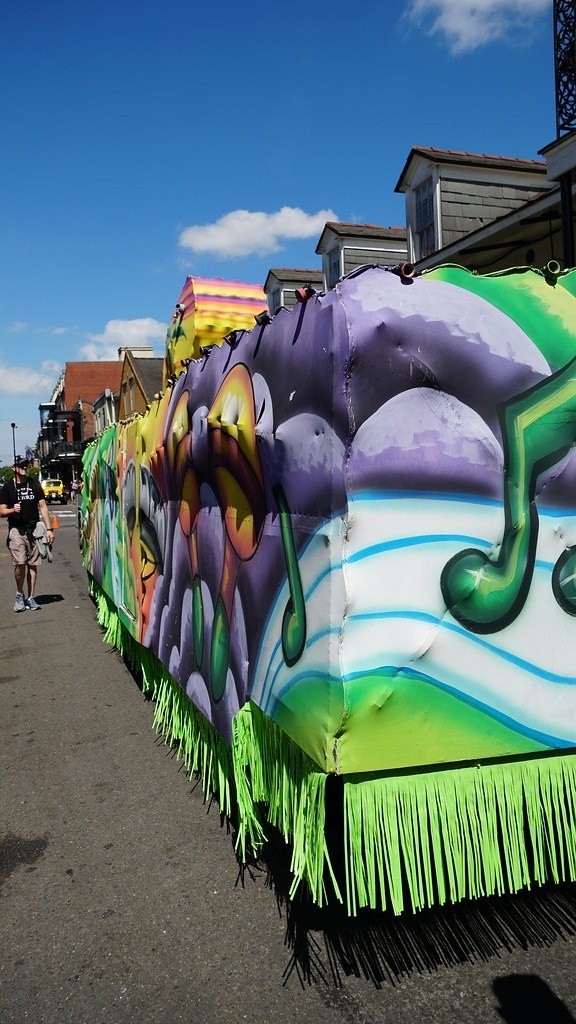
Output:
[18,464,28,468]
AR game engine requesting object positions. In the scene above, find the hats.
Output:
[9,455,30,467]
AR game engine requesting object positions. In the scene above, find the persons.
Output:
[69,479,82,501]
[0,455,55,612]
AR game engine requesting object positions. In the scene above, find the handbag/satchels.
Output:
[33,521,54,562]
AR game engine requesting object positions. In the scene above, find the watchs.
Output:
[47,528,53,532]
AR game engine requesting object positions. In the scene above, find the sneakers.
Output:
[25,598,42,611]
[14,594,25,612]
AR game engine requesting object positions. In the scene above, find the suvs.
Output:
[41,479,67,505]
